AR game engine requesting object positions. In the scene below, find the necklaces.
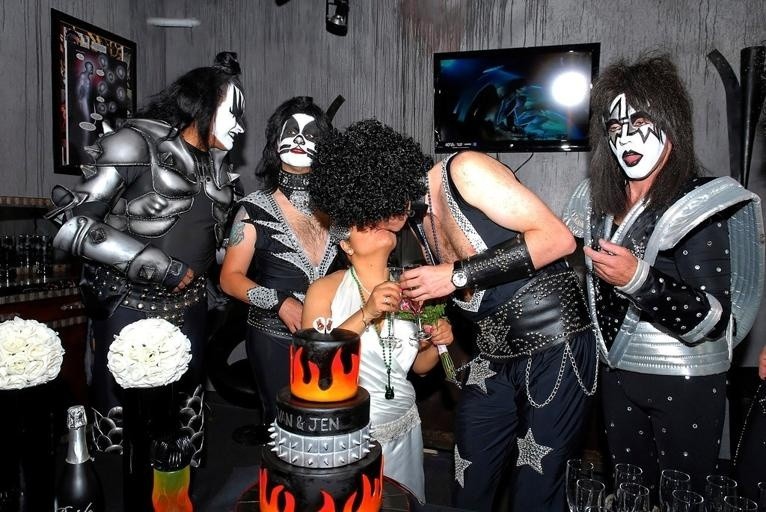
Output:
[352,270,372,297]
[350,267,394,399]
[417,172,441,265]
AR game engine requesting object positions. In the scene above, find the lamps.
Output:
[324,1,349,36]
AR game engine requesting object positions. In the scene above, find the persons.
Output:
[218,95,350,430]
[42,51,245,473]
[308,118,600,512]
[561,57,766,511]
[302,218,454,505]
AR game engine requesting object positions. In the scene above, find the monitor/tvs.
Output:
[433,42,600,152]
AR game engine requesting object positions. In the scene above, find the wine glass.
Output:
[723,495,758,511]
[381,267,402,340]
[673,490,705,512]
[566,459,595,512]
[757,482,766,511]
[705,475,737,512]
[659,470,691,512]
[403,264,432,341]
[614,463,644,505]
[575,479,605,512]
[616,483,650,512]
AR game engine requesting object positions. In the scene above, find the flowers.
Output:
[0,315,66,392]
[383,290,457,384]
[105,318,193,390]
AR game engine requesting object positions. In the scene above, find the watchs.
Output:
[450,260,470,290]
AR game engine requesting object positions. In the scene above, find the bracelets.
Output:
[360,305,368,330]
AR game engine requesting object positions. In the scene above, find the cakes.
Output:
[258,316,383,511]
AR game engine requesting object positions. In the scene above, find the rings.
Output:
[385,297,388,303]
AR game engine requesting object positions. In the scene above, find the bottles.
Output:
[1,233,51,290]
[54,405,106,512]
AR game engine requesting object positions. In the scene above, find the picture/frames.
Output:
[50,6,137,175]
